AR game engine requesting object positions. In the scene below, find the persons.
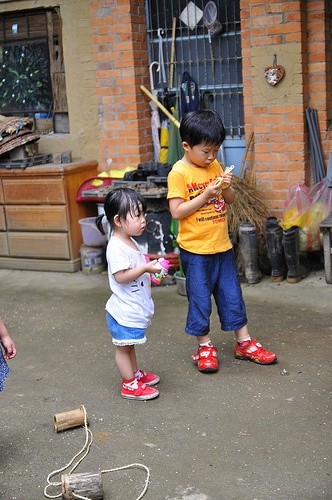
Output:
[0,318,17,393]
[94,187,163,401]
[166,109,279,373]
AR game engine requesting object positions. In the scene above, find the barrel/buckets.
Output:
[78,217,108,246]
[80,244,103,273]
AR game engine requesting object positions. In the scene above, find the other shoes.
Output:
[191,345,219,372]
[234,339,275,364]
[120,380,159,400]
[131,369,160,386]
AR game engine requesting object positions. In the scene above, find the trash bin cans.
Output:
[122,162,175,285]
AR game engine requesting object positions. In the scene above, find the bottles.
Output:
[152,260,170,285]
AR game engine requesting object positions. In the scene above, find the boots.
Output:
[238,223,262,284]
[265,226,285,282]
[282,225,307,283]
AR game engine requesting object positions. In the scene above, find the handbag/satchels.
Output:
[278,177,332,253]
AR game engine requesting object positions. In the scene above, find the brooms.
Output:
[139,84,272,248]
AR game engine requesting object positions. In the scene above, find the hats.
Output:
[0,114,40,156]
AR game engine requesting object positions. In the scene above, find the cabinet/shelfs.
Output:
[0,160,98,273]
